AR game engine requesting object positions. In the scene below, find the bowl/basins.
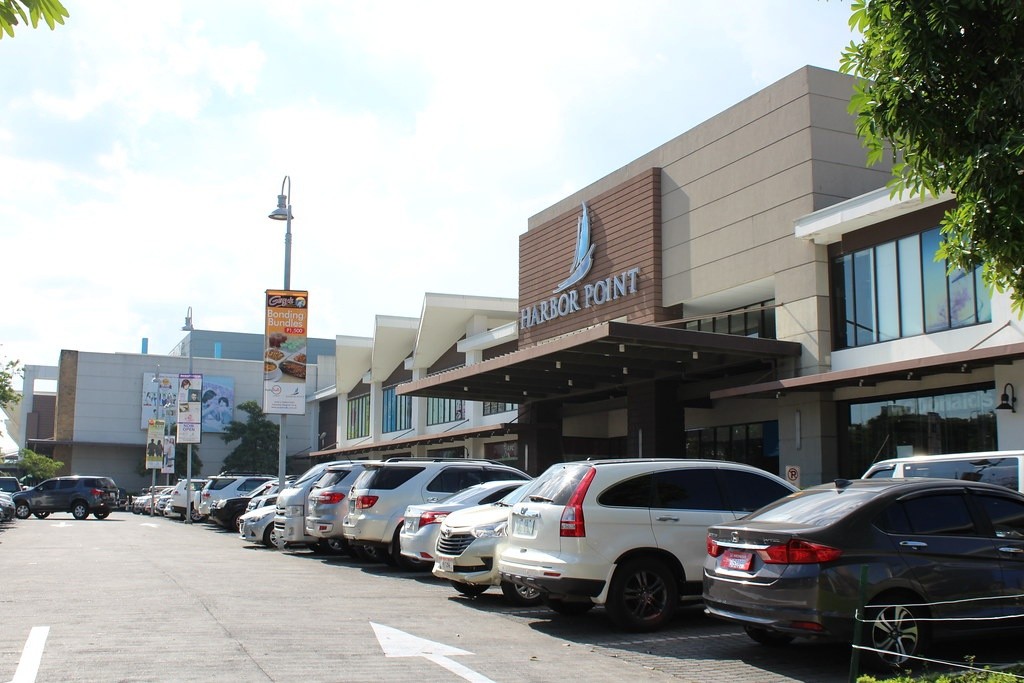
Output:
[264,359,278,379]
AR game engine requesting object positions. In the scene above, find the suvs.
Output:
[342,456,541,573]
[198,471,277,516]
[273,459,366,554]
[170,479,212,525]
[305,458,382,558]
[496,458,803,634]
[11,475,121,521]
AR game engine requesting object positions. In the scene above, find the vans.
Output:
[860,450,1024,495]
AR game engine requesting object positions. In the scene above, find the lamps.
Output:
[858,379,866,387]
[907,371,914,379]
[775,391,784,398]
[995,382,1016,414]
[961,364,969,372]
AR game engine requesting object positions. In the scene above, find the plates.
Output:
[264,347,290,364]
[287,346,306,366]
[267,368,283,382]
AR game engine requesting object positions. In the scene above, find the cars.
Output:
[0,473,46,525]
[431,475,544,609]
[399,480,535,562]
[120,484,175,516]
[702,476,1024,676]
[207,480,299,550]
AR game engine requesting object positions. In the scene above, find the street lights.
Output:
[179,306,195,524]
[149,364,162,516]
[268,173,294,489]
